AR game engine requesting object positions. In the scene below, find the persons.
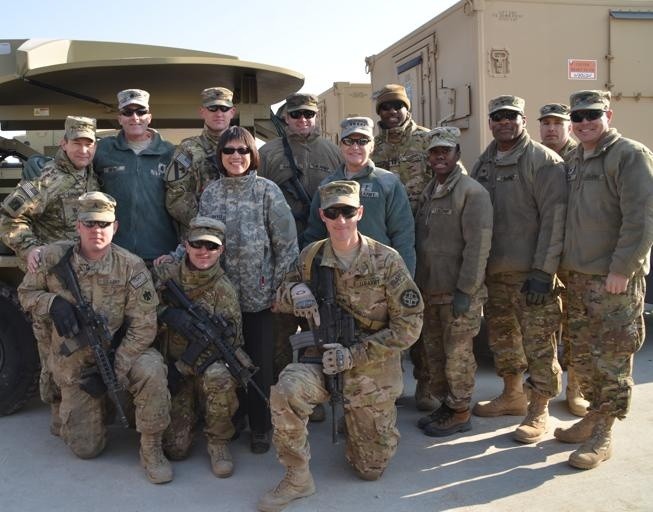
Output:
[256,93,345,238]
[522,103,591,417]
[16,191,174,485]
[553,90,652,470]
[257,179,424,511]
[161,87,235,245]
[295,117,417,282]
[197,125,300,454]
[401,126,494,439]
[366,84,469,216]
[0,115,99,436]
[147,217,248,477]
[468,95,567,445]
[20,89,179,271]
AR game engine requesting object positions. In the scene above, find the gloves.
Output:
[290,282,321,327]
[322,343,352,375]
[79,363,107,398]
[49,296,80,337]
[520,269,551,306]
[450,289,469,319]
[21,153,51,181]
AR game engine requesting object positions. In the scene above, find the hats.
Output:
[285,85,461,150]
[64,116,96,141]
[117,89,150,108]
[318,180,360,210]
[187,217,226,246]
[200,87,234,108]
[76,191,117,223]
[81,221,111,228]
[488,90,611,120]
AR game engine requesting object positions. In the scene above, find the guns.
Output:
[270,110,312,205]
[54,259,130,431]
[289,255,355,444]
[159,277,270,406]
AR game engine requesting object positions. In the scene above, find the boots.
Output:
[516,386,548,442]
[474,370,528,414]
[203,437,233,478]
[565,369,590,416]
[420,401,473,435]
[137,432,173,483]
[568,413,614,468]
[555,414,588,442]
[257,464,317,512]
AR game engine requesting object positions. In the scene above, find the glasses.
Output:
[489,110,517,121]
[570,111,605,122]
[223,147,250,154]
[289,110,315,119]
[342,137,370,146]
[322,207,357,219]
[119,106,147,116]
[207,105,229,112]
[381,101,403,110]
[187,241,219,250]
